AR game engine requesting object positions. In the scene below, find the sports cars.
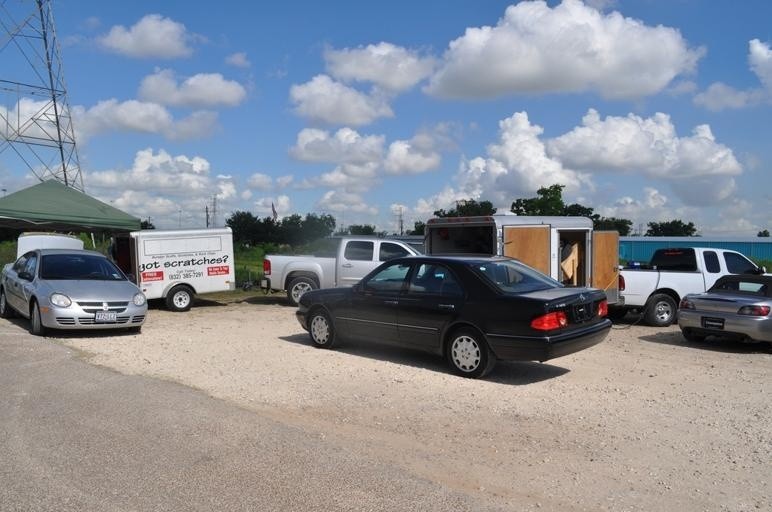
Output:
[677,274,772,343]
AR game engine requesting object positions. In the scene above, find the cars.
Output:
[1,248,149,333]
[295,253,612,380]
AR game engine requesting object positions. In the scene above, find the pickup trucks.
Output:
[260,235,426,307]
[608,248,767,327]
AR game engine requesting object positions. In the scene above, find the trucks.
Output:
[113,226,237,311]
[423,216,620,306]
